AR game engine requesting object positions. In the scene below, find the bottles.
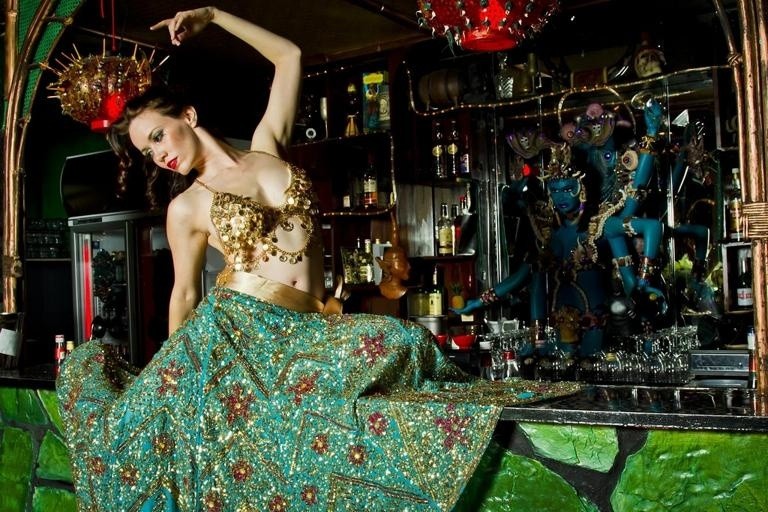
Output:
[408,264,447,317]
[492,52,513,100]
[437,195,467,257]
[745,327,758,390]
[737,257,753,309]
[634,32,668,80]
[432,119,471,178]
[363,153,378,209]
[485,320,567,380]
[727,168,743,242]
[363,238,381,284]
[54,335,75,378]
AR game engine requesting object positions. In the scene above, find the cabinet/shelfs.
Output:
[288,0,751,395]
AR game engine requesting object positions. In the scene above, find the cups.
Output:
[514,73,529,98]
[341,247,363,284]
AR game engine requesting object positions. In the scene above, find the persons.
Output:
[451,90,709,361]
[55,7,583,511]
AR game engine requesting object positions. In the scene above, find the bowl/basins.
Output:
[434,335,449,347]
[452,335,477,349]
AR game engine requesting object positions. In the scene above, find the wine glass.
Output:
[592,324,702,384]
[527,52,538,96]
[319,97,328,137]
